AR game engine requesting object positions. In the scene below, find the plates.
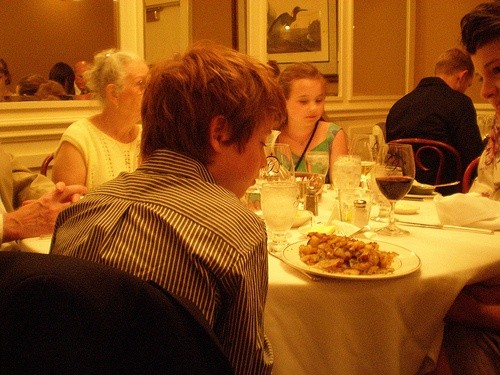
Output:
[292,209,314,228]
[283,238,421,280]
[404,191,442,199]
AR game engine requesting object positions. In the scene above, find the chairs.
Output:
[0,250,237,375]
[386,140,464,195]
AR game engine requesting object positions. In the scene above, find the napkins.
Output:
[434,189,500,230]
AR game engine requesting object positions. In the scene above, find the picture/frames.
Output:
[245,0,338,76]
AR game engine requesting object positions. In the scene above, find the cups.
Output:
[336,187,373,221]
[330,154,362,191]
[245,186,262,216]
[255,143,296,190]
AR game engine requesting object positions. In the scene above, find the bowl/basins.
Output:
[410,181,436,195]
[272,171,326,197]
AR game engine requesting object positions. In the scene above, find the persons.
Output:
[278,63,348,184]
[48,41,287,375]
[0,140,86,246]
[0,57,93,100]
[386,48,484,196]
[460,0,500,201]
[52,47,153,195]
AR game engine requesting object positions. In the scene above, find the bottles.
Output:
[352,200,368,228]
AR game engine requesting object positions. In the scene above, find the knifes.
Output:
[396,221,494,234]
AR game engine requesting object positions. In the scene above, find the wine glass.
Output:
[372,165,403,221]
[374,141,416,237]
[259,182,299,255]
[347,134,379,188]
[304,150,330,194]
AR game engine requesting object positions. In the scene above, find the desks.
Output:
[10,183,500,375]
